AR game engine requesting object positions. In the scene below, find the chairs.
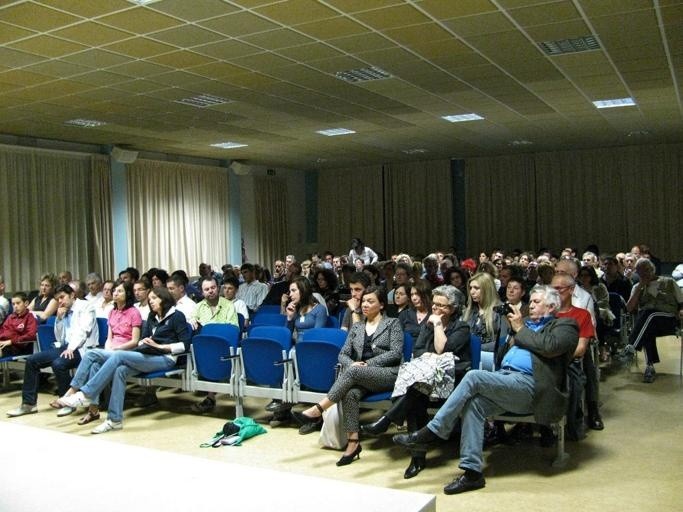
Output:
[427,335,571,468]
[0,315,108,396]
[359,332,413,410]
[637,308,682,366]
[127,306,349,423]
[579,291,637,425]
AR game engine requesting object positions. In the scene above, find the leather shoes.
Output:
[392,429,434,450]
[404,458,428,478]
[360,414,391,438]
[443,474,487,495]
[587,408,603,429]
[644,366,656,383]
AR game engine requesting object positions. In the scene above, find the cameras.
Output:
[493,303,513,315]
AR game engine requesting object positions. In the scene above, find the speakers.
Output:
[229,160,251,176]
[112,145,139,163]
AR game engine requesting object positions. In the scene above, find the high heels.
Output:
[293,404,323,426]
[335,439,362,466]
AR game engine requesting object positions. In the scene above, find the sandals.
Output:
[77,411,100,426]
[49,398,68,408]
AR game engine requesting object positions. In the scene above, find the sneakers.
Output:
[60,391,99,409]
[91,420,123,434]
[6,404,38,417]
[191,397,216,413]
[56,406,77,416]
[265,399,322,433]
